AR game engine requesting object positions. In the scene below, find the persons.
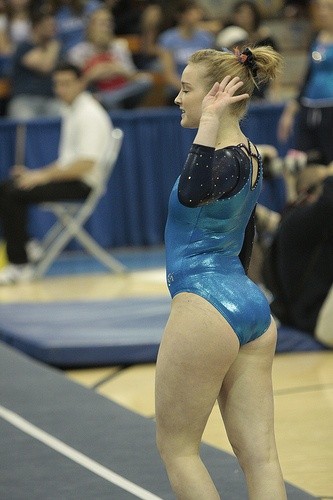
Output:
[0,0,333,158]
[151,46,293,499]
[0,62,112,286]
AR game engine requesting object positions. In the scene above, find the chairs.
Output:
[18,128,132,282]
